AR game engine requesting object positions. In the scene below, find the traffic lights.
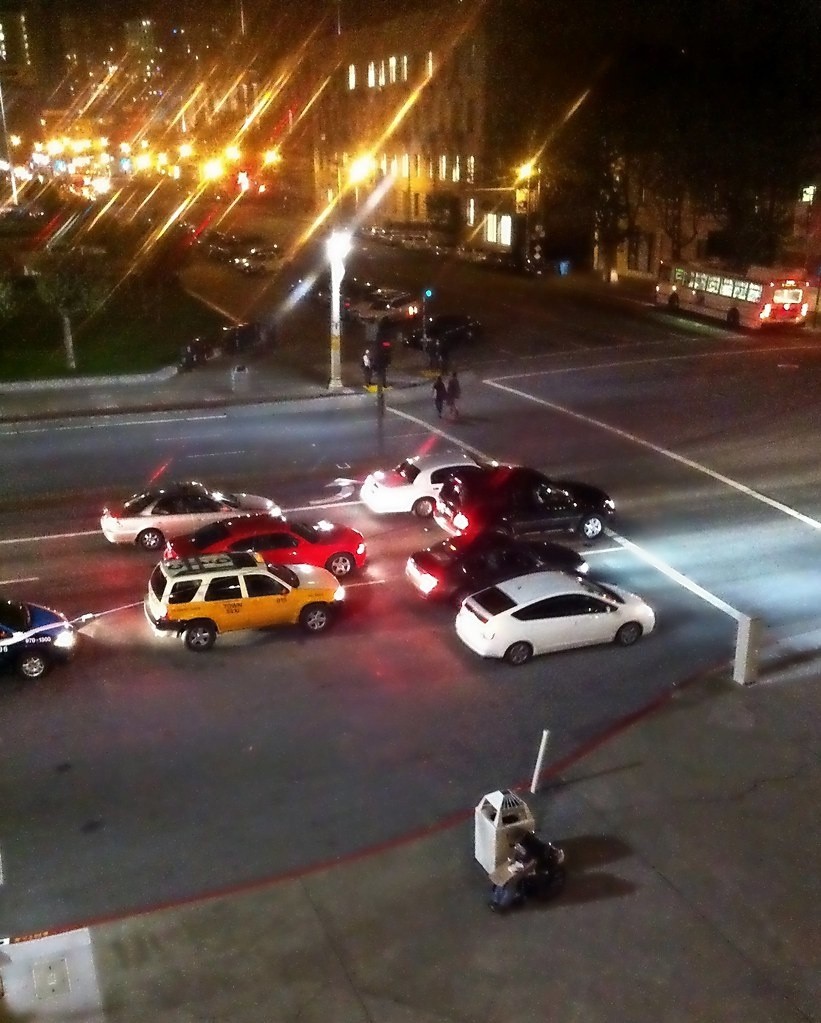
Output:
[423,285,436,301]
[377,324,393,364]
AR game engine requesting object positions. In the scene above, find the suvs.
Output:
[143,548,348,652]
[435,466,617,541]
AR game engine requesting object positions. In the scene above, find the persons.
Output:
[446,372,462,417]
[361,347,391,388]
[432,377,447,418]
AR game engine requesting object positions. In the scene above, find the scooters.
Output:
[490,827,566,915]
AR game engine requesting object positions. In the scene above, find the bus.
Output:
[655,256,812,330]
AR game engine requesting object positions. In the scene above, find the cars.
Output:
[407,310,480,352]
[405,531,588,600]
[1,599,77,683]
[164,513,369,579]
[357,448,510,519]
[456,570,658,665]
[98,482,287,548]
[334,212,519,273]
[292,263,427,326]
[34,160,314,277]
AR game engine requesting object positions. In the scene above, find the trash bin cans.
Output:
[230,364,248,393]
[474,788,536,874]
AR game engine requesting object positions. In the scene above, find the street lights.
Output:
[326,230,351,391]
[350,160,364,225]
[522,165,532,255]
